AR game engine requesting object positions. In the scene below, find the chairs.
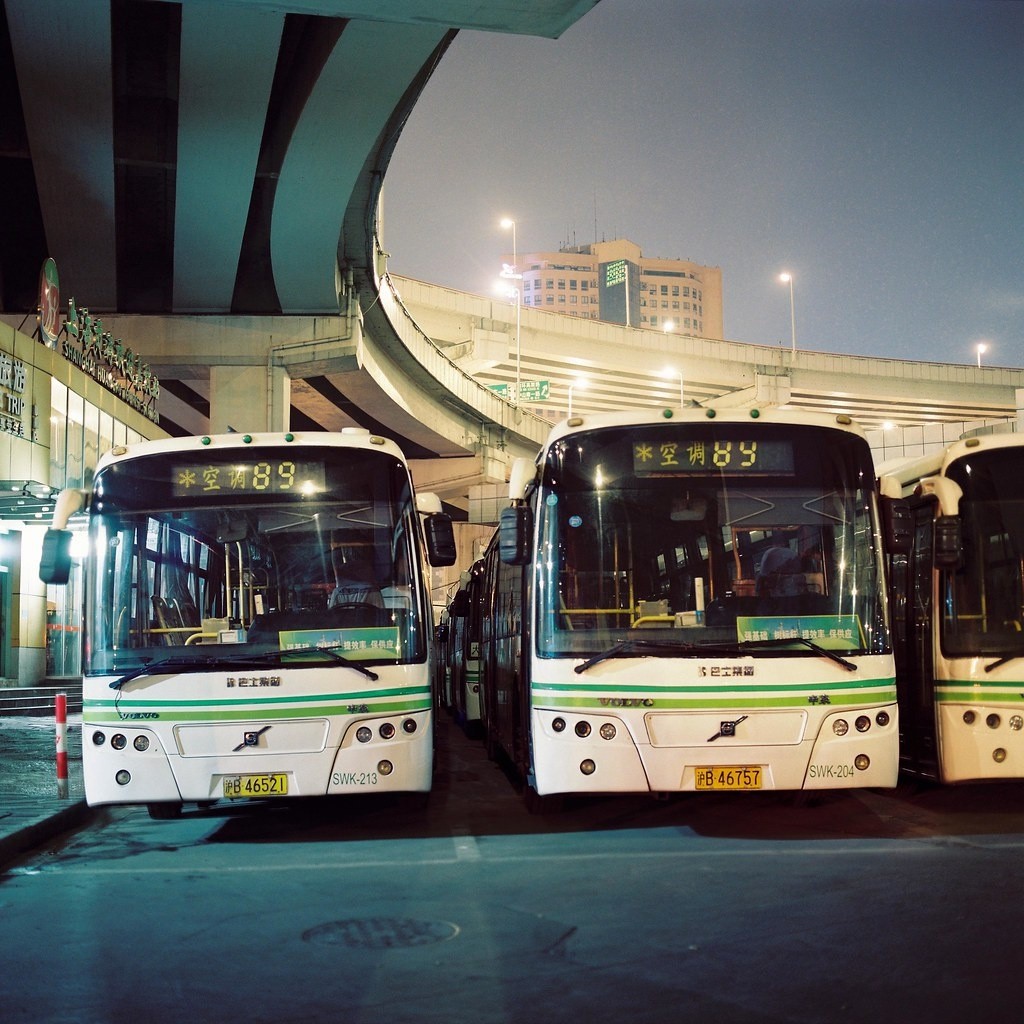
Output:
[329,585,384,611]
[587,546,804,624]
[150,597,198,645]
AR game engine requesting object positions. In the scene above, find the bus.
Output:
[40,425,457,819]
[435,408,1024,796]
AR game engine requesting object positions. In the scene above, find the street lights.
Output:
[662,366,683,409]
[569,381,589,420]
[977,345,985,369]
[780,273,796,361]
[500,219,521,403]
